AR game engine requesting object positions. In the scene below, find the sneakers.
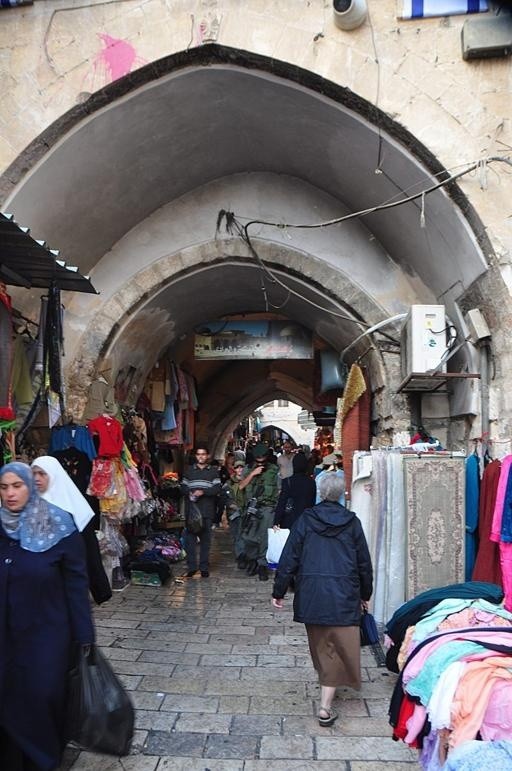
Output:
[186,569,199,577]
[238,559,268,580]
[201,571,210,578]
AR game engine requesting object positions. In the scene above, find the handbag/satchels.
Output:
[360,605,381,646]
[284,498,295,514]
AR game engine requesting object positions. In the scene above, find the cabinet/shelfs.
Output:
[147,439,188,532]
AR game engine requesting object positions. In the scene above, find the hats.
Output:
[322,454,338,465]
[233,461,245,469]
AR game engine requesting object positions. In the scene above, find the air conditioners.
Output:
[398,302,449,393]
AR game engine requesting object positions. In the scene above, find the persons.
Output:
[0,460,95,771]
[182,440,337,582]
[29,456,113,605]
[271,474,373,726]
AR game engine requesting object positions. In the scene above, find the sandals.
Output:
[318,705,338,727]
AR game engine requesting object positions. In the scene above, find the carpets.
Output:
[343,447,469,633]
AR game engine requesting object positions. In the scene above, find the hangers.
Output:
[52,415,91,434]
[99,407,115,422]
[471,437,510,469]
